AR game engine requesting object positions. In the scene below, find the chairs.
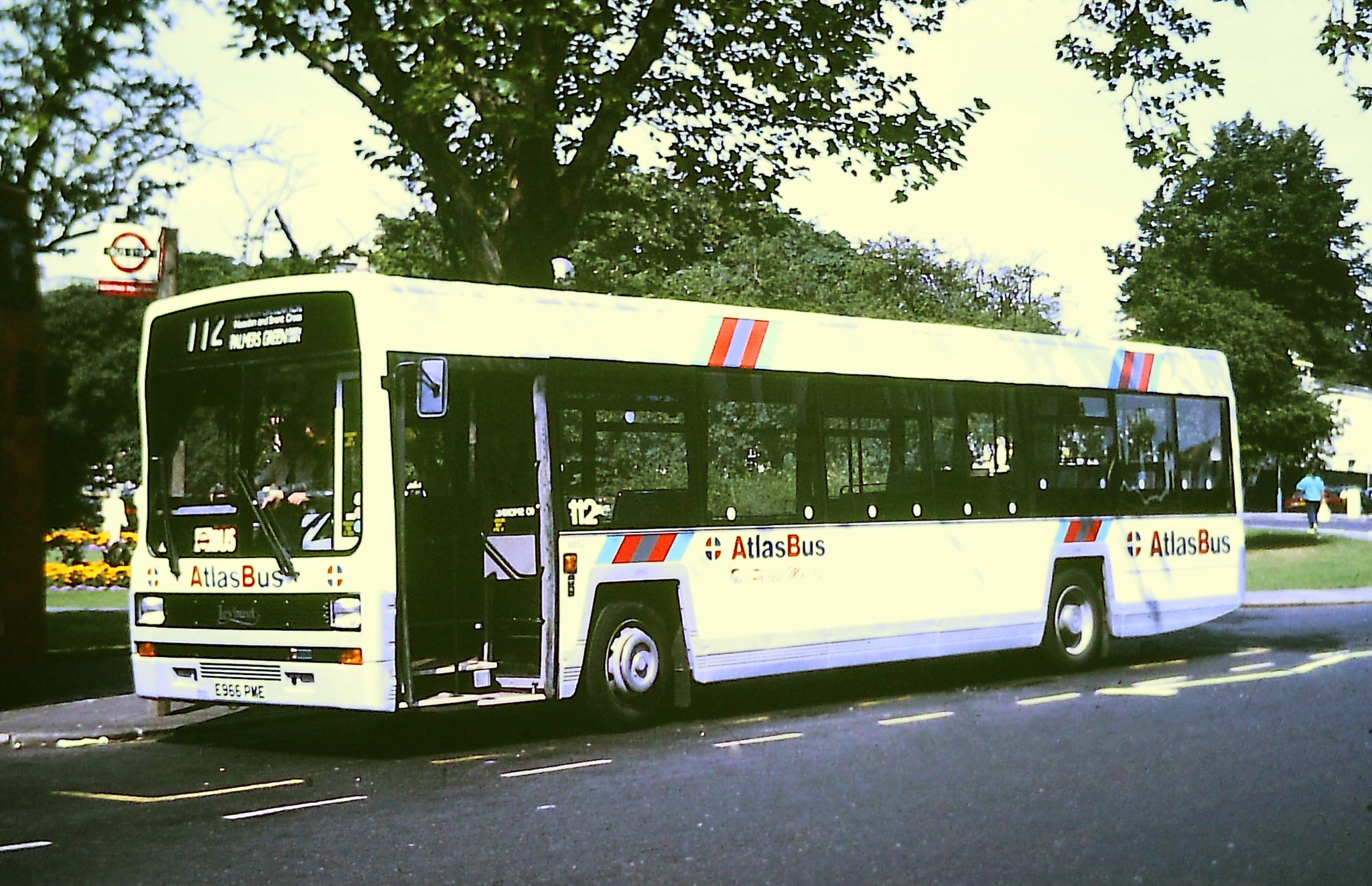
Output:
[607,491,692,529]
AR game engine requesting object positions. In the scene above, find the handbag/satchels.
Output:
[1316,502,1331,523]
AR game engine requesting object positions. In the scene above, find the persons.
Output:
[99,489,128,547]
[253,418,334,509]
[1296,463,1325,534]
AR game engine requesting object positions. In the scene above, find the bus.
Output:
[125,272,1253,735]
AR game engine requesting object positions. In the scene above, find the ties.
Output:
[285,463,296,484]
